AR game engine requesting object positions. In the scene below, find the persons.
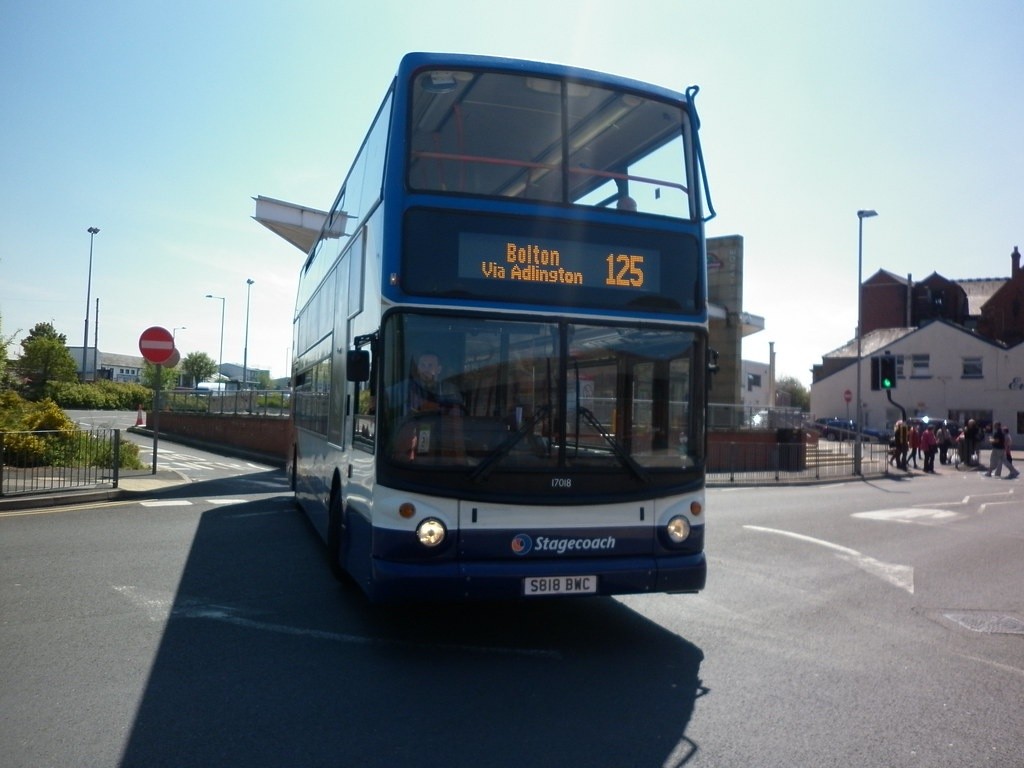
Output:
[616,194,638,211]
[384,350,464,418]
[888,418,1020,480]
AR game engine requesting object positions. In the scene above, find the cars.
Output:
[826,419,884,444]
[812,417,840,435]
[753,411,771,427]
[907,417,958,435]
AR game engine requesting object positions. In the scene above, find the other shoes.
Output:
[1005,471,1019,480]
[984,473,991,476]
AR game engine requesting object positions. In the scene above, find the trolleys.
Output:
[955,440,964,469]
[946,439,954,464]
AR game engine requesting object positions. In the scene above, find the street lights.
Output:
[81,227,100,384]
[205,294,225,395]
[854,209,879,474]
[243,279,254,389]
[173,327,186,340]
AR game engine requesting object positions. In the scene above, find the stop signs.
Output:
[844,390,852,402]
[139,326,175,365]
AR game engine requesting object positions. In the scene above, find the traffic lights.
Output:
[881,355,895,389]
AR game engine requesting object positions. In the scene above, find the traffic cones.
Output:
[134,404,143,426]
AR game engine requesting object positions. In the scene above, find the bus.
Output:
[286,52,719,601]
